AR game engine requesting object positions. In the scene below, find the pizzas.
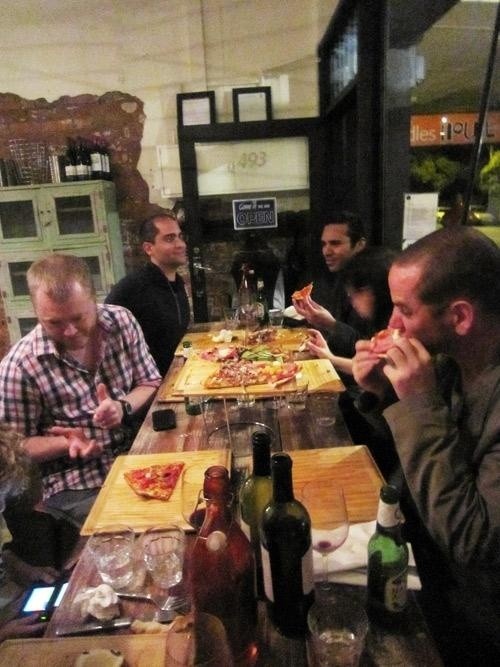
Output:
[292,282,314,300]
[122,462,185,501]
[370,328,400,359]
[201,357,304,388]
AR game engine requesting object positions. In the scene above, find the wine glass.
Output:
[302,479,349,592]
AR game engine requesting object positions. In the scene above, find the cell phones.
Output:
[21,586,56,612]
[152,408,177,431]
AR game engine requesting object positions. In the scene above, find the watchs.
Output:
[114,399,132,424]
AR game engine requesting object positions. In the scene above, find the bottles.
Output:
[238,264,268,324]
[190,432,315,665]
[63,135,113,183]
[366,485,411,622]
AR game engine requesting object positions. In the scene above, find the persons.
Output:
[0,254,160,566]
[353,221,500,666]
[0,424,60,640]
[306,243,397,476]
[291,210,383,411]
[102,208,193,384]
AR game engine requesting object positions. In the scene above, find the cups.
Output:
[282,379,339,428]
[235,392,257,406]
[224,307,238,330]
[185,395,204,415]
[269,310,284,327]
[308,594,370,666]
[85,522,186,587]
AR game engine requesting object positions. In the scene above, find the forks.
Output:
[82,584,188,610]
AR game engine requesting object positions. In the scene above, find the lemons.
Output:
[72,647,123,667]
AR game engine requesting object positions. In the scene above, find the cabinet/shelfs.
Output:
[2,180,127,345]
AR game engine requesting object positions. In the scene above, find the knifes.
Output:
[56,609,186,636]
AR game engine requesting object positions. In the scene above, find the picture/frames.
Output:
[175,85,272,125]
